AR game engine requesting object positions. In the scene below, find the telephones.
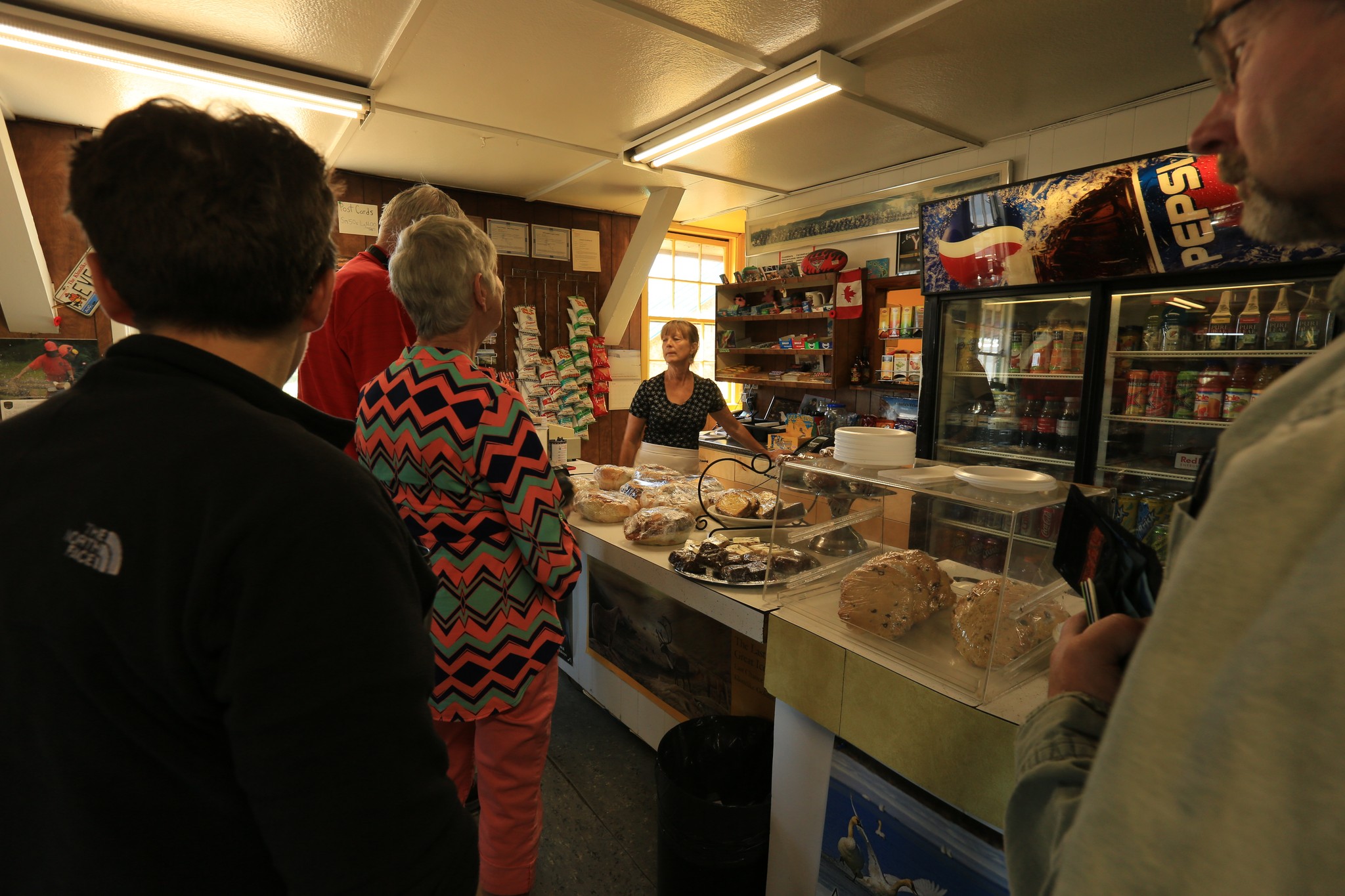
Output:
[716,410,752,427]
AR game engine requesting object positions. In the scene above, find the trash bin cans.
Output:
[654,715,775,896]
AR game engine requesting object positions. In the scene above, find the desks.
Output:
[766,560,1087,896]
[553,459,939,751]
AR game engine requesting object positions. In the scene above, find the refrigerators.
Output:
[906,145,1345,601]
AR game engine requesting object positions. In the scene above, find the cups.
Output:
[549,438,567,467]
[805,290,826,306]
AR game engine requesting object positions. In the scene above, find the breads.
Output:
[952,578,1071,669]
[564,447,872,583]
[838,549,957,641]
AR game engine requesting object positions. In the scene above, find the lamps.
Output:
[617,50,865,175]
[0,0,376,132]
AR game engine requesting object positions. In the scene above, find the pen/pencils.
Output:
[1183,446,1215,518]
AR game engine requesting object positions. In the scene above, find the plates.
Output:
[706,504,808,528]
[672,538,821,586]
[953,464,1058,494]
[833,426,916,470]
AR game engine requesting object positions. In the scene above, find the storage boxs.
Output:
[762,456,1117,706]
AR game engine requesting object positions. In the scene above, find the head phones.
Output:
[71,349,78,355]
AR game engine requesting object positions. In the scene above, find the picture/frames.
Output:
[895,229,921,276]
[745,159,1014,257]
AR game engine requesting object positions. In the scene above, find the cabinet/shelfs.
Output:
[876,327,923,385]
[715,272,849,390]
[699,446,912,550]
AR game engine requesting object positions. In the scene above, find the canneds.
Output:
[1110,485,1189,563]
[1122,368,1199,420]
[1124,153,1260,272]
[936,502,1062,575]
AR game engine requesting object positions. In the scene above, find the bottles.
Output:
[824,403,848,440]
[945,282,1340,462]
[816,400,837,413]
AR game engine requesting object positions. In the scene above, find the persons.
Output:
[355,214,582,896]
[0,99,469,896]
[298,182,469,462]
[1003,0,1345,896]
[618,320,794,476]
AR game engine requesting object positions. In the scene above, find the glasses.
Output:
[1189,0,1255,92]
